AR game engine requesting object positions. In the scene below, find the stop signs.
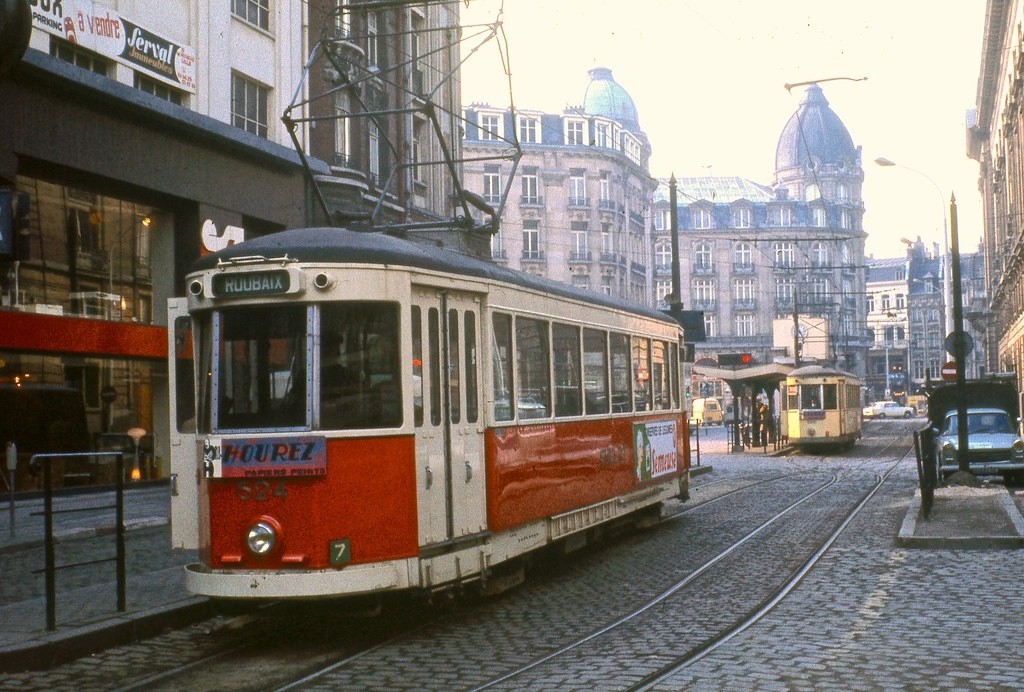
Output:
[941,362,958,382]
[101,387,116,401]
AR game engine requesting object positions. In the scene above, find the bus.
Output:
[165,224,691,613]
[779,364,862,451]
[0,387,91,492]
[899,396,929,417]
[95,433,153,470]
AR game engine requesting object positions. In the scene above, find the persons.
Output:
[756,401,769,446]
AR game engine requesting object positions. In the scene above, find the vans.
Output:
[693,398,723,426]
[723,404,744,426]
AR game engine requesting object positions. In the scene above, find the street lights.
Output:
[912,279,955,363]
[882,343,892,397]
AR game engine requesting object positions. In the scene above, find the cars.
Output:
[863,401,915,418]
[931,407,1024,483]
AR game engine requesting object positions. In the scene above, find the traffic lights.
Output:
[686,388,689,397]
[717,353,751,365]
